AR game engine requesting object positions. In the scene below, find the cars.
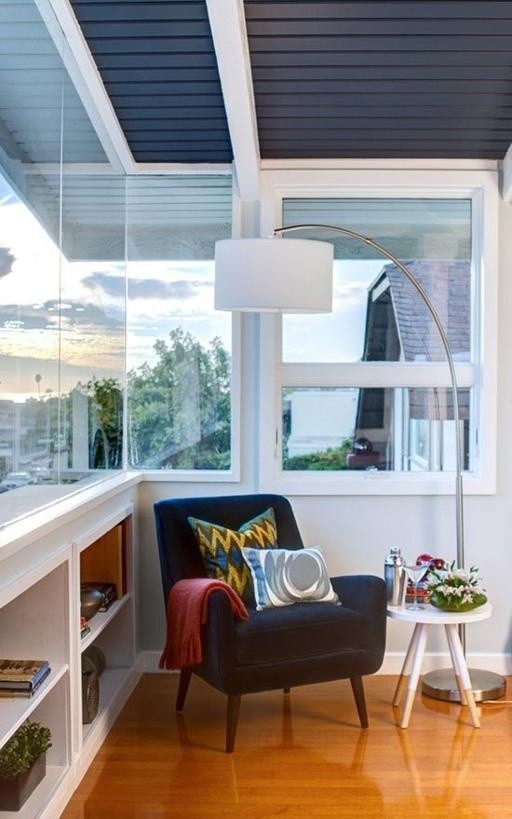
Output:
[0,466,49,494]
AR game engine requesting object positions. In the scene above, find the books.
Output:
[0,659,51,698]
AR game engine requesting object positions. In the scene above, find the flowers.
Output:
[423,560,489,610]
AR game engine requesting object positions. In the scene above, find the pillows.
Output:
[184,507,343,611]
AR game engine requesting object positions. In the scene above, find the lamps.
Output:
[214,223,509,702]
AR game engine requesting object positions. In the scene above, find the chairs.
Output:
[150,493,389,753]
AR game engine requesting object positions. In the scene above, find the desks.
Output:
[387,601,493,729]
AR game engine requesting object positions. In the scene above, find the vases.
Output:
[429,592,488,613]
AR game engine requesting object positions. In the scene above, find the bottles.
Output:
[383,546,406,605]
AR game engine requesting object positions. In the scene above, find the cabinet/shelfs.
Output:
[0,470,142,819]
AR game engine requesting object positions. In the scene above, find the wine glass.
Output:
[401,561,431,610]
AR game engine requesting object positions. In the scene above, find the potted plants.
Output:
[0,714,54,811]
